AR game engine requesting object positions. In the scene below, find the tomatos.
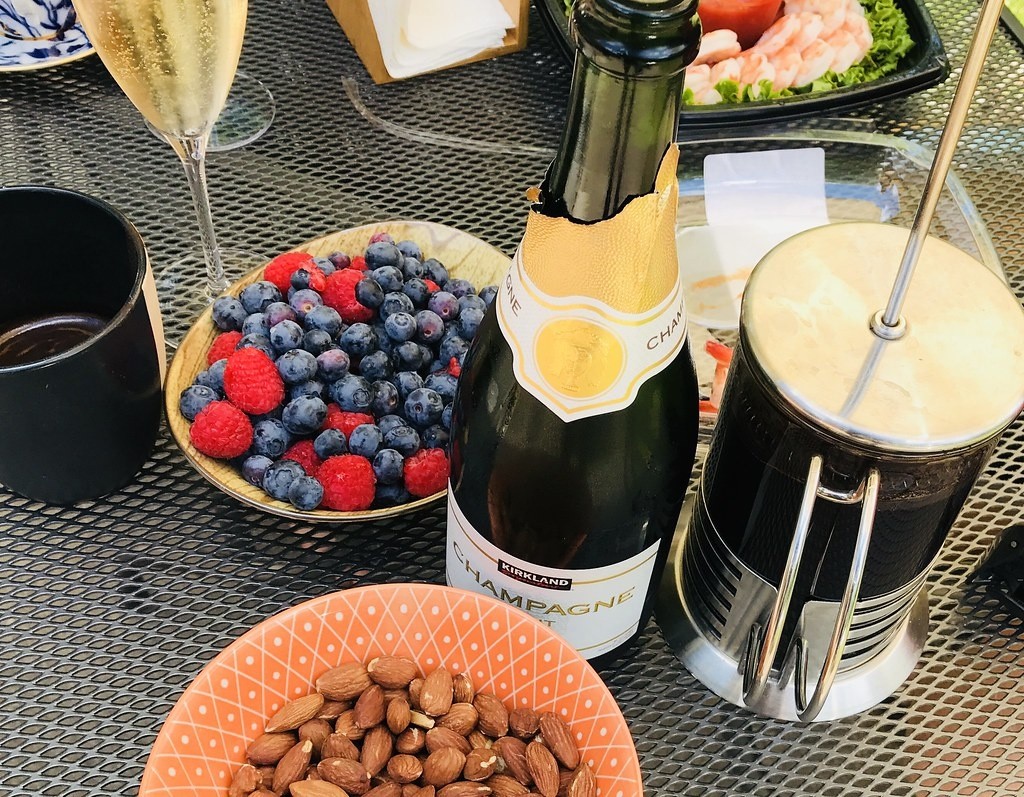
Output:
[696,0,784,50]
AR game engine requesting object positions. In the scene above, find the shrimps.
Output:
[685,1,873,105]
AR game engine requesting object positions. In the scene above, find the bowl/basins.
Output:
[163,218,511,521]
[135,583,642,797]
[672,131,1011,449]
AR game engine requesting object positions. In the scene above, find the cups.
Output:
[0,185,167,507]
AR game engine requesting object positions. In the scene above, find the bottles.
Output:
[440,0,705,669]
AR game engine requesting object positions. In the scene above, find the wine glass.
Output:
[68,1,272,346]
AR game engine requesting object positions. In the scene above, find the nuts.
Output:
[227,654,598,797]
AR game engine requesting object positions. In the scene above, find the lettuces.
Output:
[682,0,917,108]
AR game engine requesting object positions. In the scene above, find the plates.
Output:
[541,0,953,130]
